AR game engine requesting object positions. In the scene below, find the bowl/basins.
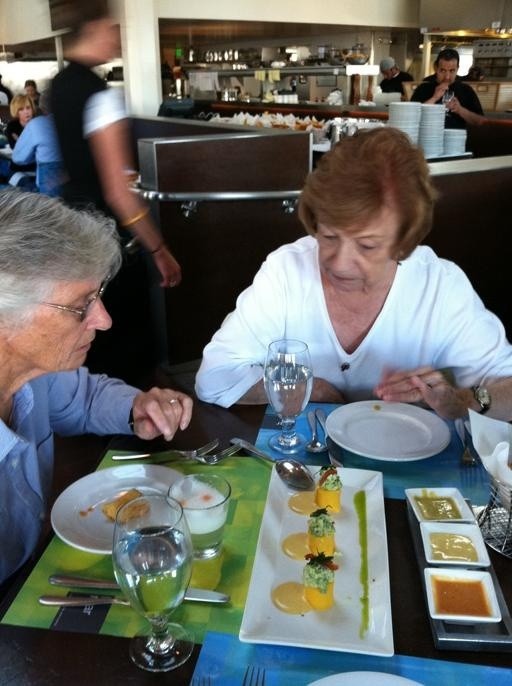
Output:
[345,56,369,65]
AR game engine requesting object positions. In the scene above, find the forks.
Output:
[112,435,244,467]
[453,414,480,469]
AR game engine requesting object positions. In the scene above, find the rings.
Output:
[169,398,178,404]
[426,382,433,389]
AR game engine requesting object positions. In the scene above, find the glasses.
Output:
[41,279,107,323]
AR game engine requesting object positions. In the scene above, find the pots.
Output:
[326,47,346,65]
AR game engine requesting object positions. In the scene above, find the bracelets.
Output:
[149,240,167,255]
[119,207,152,228]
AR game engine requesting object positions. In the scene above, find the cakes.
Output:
[316,470,340,513]
[303,555,335,610]
[307,508,336,557]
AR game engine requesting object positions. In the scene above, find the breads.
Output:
[102,487,151,525]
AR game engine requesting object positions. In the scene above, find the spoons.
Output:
[303,408,324,455]
[230,435,315,489]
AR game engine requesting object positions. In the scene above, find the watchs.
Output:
[472,384,490,416]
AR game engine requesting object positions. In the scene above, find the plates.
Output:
[389,100,467,155]
[404,486,503,626]
[325,399,453,462]
[50,462,182,552]
[239,461,396,658]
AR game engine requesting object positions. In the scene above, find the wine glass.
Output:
[262,337,313,457]
[108,486,199,673]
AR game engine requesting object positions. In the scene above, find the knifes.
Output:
[315,407,345,468]
[47,573,231,607]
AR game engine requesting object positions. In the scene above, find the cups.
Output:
[441,90,456,113]
[166,471,234,562]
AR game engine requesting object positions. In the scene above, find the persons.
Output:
[46,6,185,384]
[409,48,489,130]
[379,55,417,95]
[193,126,511,427]
[1,78,73,202]
[0,184,196,579]
